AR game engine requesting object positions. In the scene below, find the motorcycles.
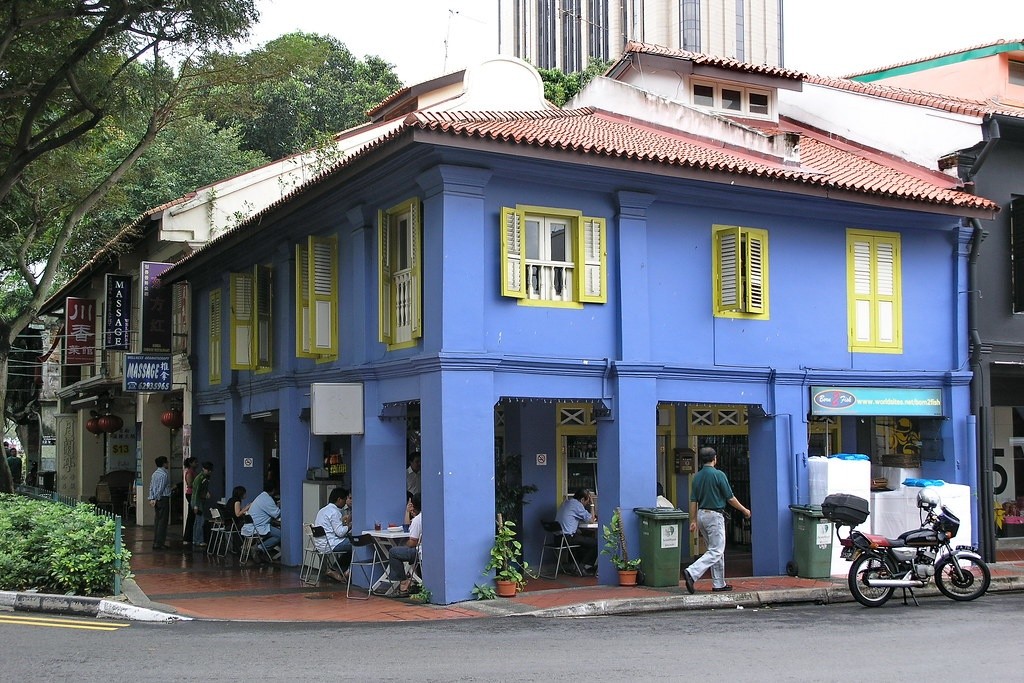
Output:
[822,487,991,607]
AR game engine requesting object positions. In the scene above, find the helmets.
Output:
[917,488,938,508]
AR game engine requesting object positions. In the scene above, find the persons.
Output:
[683,447,750,594]
[147,456,171,549]
[406,451,421,494]
[7,448,22,487]
[227,485,251,525]
[388,491,422,597]
[313,488,352,581]
[183,456,214,546]
[585,489,598,521]
[657,482,674,508]
[3,441,10,458]
[552,488,598,576]
[241,481,281,564]
[338,490,352,522]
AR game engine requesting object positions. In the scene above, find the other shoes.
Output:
[582,567,596,575]
[327,569,343,581]
[390,589,410,597]
[252,544,265,561]
[193,541,208,546]
[153,543,169,548]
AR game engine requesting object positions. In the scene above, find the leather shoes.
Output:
[711,584,733,591]
[682,570,696,594]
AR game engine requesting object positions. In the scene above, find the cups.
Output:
[221,498,226,503]
[374,521,382,532]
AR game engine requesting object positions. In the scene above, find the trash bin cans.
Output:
[633,508,690,587]
[788,504,834,577]
[36,470,57,495]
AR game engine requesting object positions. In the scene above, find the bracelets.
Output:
[590,504,595,506]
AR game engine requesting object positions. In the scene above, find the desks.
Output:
[577,522,600,533]
[362,528,423,596]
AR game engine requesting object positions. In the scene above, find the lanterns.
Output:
[86,415,103,437]
[98,413,124,435]
[161,408,183,431]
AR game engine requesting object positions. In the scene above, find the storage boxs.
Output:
[881,453,920,468]
[821,493,870,528]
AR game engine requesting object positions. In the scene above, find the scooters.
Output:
[27,459,38,486]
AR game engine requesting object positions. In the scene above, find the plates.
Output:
[387,528,402,532]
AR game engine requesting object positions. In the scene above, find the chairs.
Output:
[406,533,423,595]
[538,518,583,578]
[301,522,327,581]
[216,512,244,558]
[206,507,221,554]
[231,512,272,565]
[305,524,347,585]
[345,532,395,601]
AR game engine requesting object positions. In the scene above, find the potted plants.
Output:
[599,506,643,584]
[479,512,537,597]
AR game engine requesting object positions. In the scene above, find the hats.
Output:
[202,461,214,470]
